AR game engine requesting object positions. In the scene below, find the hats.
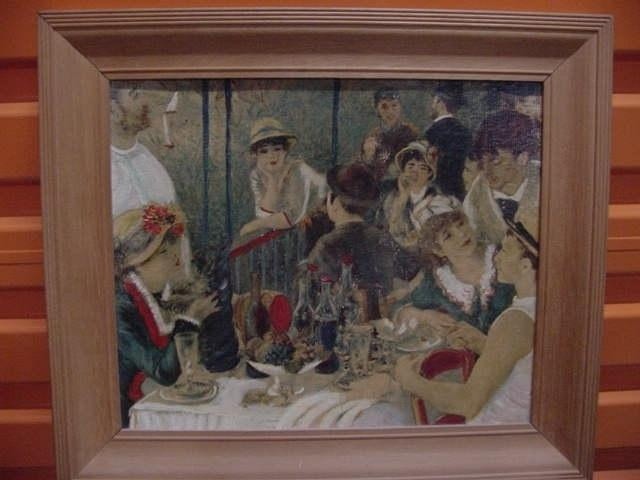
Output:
[502,211,540,257]
[326,164,384,208]
[247,118,298,156]
[112,203,183,282]
[396,141,437,184]
[409,193,462,231]
[431,81,466,105]
[500,81,543,97]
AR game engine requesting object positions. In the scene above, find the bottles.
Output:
[293,263,359,374]
[246,273,268,342]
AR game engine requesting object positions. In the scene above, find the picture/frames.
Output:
[38,6,615,480]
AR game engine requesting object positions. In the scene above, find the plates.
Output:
[394,338,442,352]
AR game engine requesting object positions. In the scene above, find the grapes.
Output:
[263,341,315,366]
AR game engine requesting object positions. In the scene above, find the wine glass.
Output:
[173,331,198,392]
